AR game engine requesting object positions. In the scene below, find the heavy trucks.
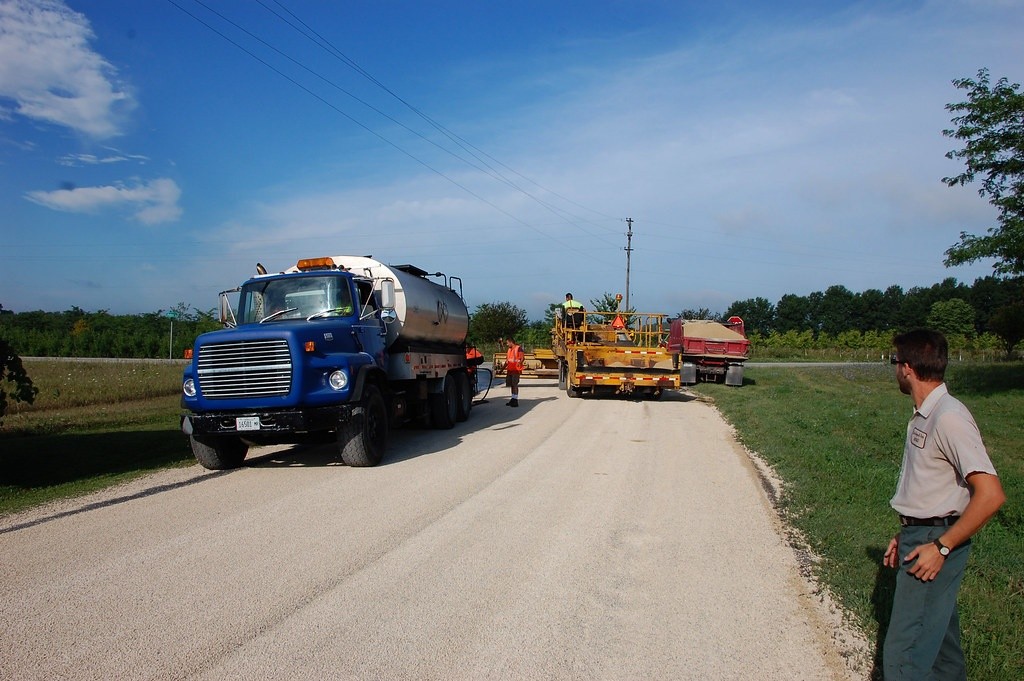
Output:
[174,253,480,471]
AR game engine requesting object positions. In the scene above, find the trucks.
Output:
[660,317,752,387]
[549,306,684,400]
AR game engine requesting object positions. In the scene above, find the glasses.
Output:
[890,359,912,368]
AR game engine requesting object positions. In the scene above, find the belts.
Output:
[898,514,960,526]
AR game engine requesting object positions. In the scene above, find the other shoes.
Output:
[511,399,518,407]
[506,398,514,406]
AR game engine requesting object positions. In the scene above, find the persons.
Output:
[334,289,353,317]
[500,337,524,407]
[562,293,586,329]
[466,344,484,404]
[880,328,1005,681]
[270,304,280,320]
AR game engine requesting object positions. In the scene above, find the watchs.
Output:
[934,538,950,559]
